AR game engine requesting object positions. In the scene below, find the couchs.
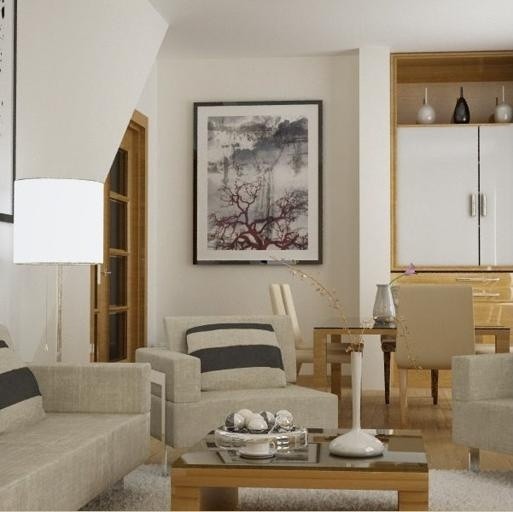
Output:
[0,325,151,503]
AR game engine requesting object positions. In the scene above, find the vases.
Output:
[372,284,395,321]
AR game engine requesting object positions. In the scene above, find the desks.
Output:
[313,321,512,402]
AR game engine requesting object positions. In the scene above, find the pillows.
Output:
[184,324,288,392]
[0,340,44,433]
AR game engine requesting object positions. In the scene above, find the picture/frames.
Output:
[192,100,325,266]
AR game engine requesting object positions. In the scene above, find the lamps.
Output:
[13,179,103,363]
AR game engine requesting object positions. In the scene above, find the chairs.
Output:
[270,283,353,379]
[449,353,511,472]
[399,283,477,419]
[136,316,338,450]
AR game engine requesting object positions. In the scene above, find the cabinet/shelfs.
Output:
[392,122,510,272]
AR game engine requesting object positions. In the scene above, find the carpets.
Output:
[82,464,513,507]
